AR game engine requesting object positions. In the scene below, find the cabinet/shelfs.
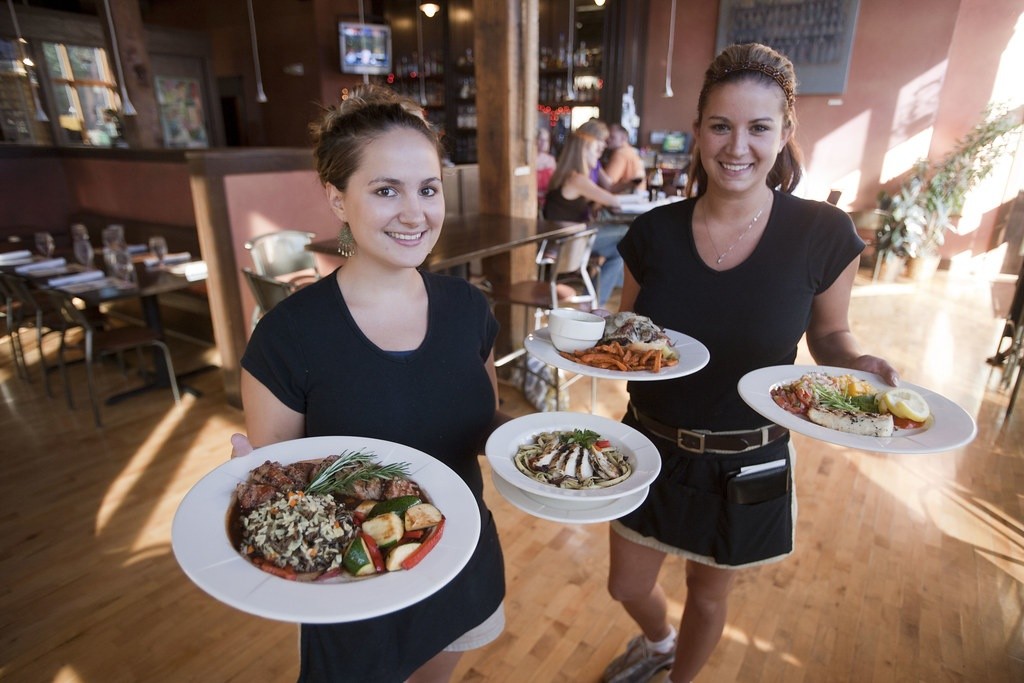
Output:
[370,0,649,166]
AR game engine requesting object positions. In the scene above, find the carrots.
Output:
[354,511,446,571]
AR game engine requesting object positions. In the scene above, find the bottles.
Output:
[539,36,601,103]
[393,49,475,126]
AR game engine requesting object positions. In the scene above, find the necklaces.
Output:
[701,191,770,264]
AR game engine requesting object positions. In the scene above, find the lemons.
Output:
[885,388,930,423]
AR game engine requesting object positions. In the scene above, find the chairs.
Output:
[242,227,606,333]
[0,272,182,428]
[851,207,897,284]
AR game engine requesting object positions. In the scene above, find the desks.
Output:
[605,208,640,224]
[305,212,587,406]
[0,240,218,406]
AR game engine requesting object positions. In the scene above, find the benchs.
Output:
[53,211,215,345]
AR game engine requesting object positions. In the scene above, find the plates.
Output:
[737,364,978,455]
[170,435,481,624]
[523,326,710,380]
[485,410,661,501]
[490,469,650,522]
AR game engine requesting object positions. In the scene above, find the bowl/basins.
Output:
[548,308,606,353]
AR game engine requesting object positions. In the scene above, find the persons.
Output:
[538,117,648,306]
[588,41,900,683]
[229,80,517,683]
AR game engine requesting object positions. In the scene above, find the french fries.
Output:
[560,341,682,373]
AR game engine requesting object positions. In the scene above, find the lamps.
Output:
[7,0,267,122]
[659,0,676,97]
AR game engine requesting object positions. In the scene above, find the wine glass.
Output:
[35,223,168,288]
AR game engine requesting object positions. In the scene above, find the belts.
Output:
[628,402,787,456]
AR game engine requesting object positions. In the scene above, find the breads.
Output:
[808,404,895,437]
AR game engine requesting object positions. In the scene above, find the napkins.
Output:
[0,249,31,264]
[47,269,102,286]
[145,252,190,266]
[14,257,66,272]
[105,245,147,254]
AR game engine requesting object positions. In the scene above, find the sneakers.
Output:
[603,628,679,683]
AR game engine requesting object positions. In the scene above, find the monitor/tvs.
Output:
[337,21,394,76]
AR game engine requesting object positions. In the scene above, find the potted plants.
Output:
[867,98,1021,285]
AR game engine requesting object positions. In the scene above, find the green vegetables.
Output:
[561,427,602,450]
[814,386,868,413]
[304,447,414,495]
